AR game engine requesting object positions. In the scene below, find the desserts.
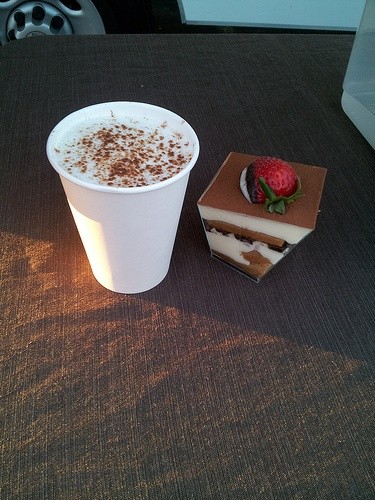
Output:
[197,151,328,282]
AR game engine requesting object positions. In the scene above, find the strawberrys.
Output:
[239,156,303,215]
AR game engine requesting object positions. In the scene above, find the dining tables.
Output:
[1,33,375,500]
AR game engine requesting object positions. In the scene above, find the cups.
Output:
[45,100,200,295]
